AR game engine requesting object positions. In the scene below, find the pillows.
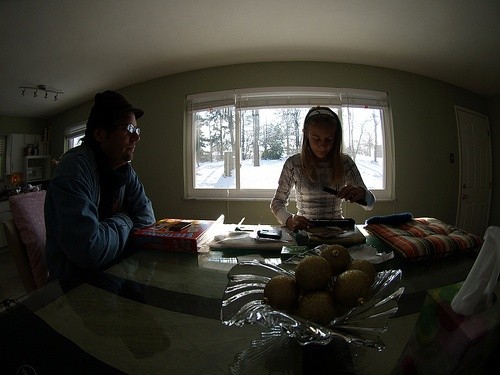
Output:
[363,217,483,261]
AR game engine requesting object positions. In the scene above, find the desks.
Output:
[1,222,476,375]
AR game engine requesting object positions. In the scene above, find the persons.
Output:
[270,106,376,232]
[45,89,155,277]
[68,249,170,360]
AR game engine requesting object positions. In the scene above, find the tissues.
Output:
[399,221,500,375]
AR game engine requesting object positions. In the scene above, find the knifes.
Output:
[308,181,345,199]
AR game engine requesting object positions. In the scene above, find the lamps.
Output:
[17,85,64,102]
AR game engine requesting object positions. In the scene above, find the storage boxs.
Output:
[136,217,217,253]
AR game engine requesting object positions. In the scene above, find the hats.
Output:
[86,89,144,129]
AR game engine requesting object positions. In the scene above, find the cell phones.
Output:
[169,222,191,231]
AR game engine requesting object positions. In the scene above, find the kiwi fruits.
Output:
[264,244,370,326]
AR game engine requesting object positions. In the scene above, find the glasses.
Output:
[116,122,141,135]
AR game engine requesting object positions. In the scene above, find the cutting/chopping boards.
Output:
[304,225,366,244]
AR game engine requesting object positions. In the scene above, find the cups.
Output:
[26,148,32,155]
[28,144,33,151]
[34,149,37,155]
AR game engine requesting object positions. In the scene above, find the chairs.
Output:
[3,189,57,294]
